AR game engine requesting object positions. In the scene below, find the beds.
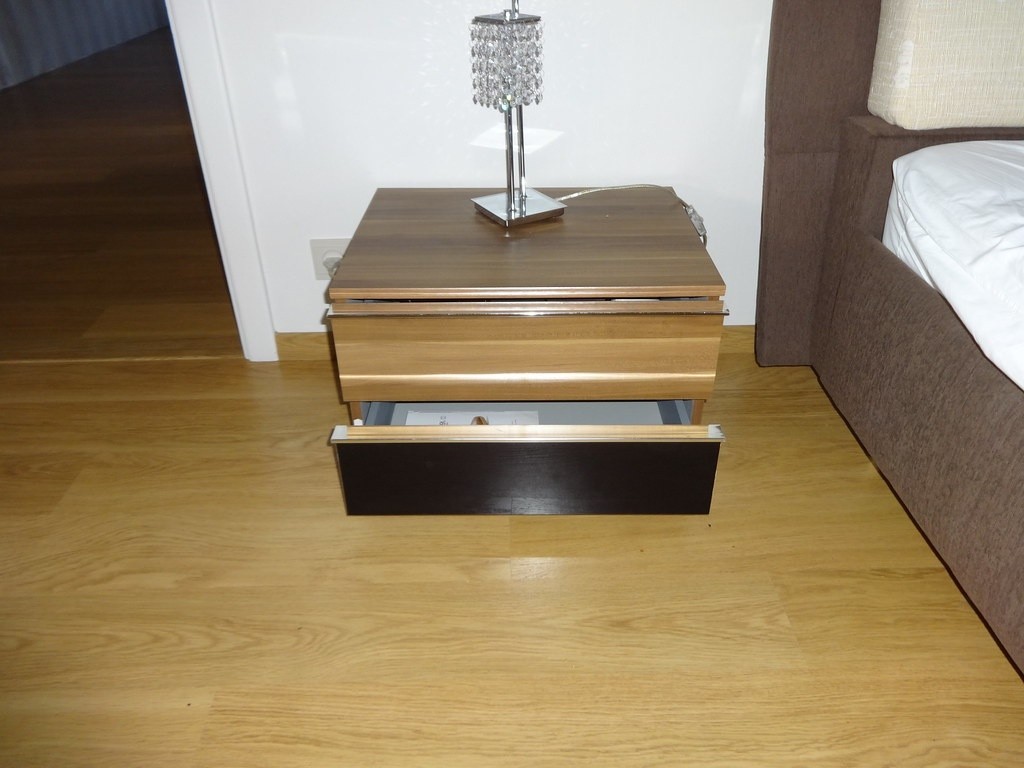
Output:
[753,0,1024,680]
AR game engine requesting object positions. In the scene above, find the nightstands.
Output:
[323,187,729,518]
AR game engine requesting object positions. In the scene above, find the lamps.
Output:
[469,0,567,229]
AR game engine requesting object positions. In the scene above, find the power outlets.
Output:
[310,239,352,281]
